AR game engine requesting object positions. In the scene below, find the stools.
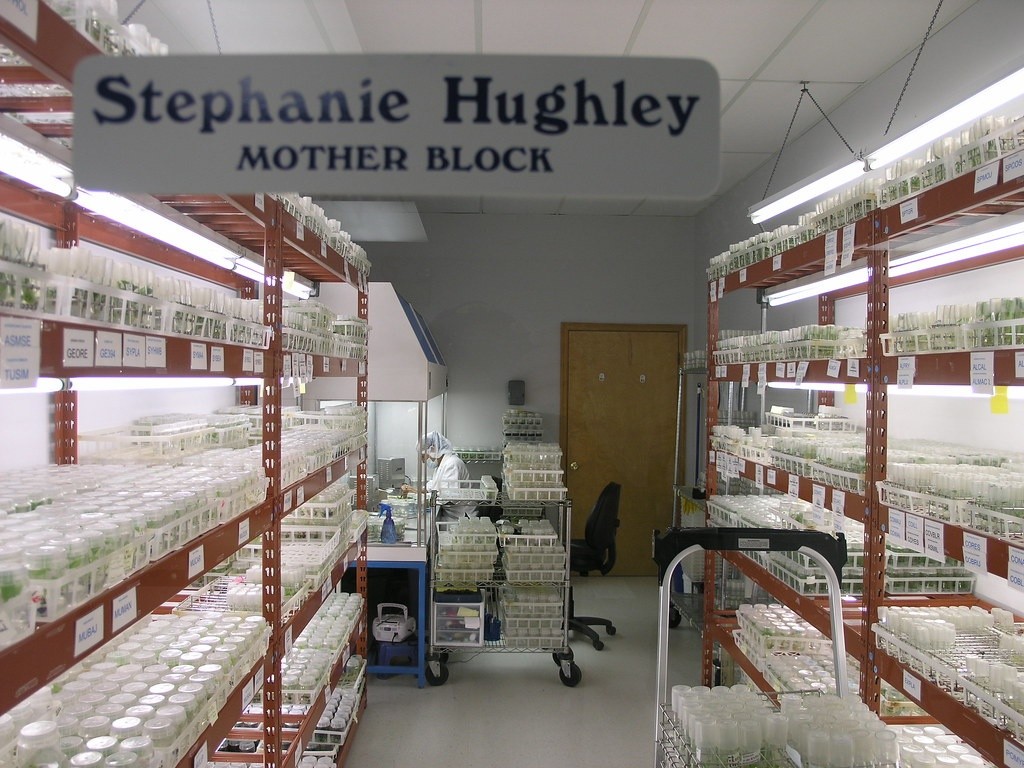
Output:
[371,635,420,679]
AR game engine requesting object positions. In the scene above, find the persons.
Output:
[400,430,479,531]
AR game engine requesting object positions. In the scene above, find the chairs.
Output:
[564,481,622,651]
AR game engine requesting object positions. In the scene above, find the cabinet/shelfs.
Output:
[425,490,582,687]
[670,116,1024,768]
[0,0,366,768]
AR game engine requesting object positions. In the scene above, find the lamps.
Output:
[868,0,1024,173]
[231,247,315,300]
[747,80,867,225]
[756,258,868,308]
[0,112,78,200]
[68,164,244,271]
[887,208,1024,278]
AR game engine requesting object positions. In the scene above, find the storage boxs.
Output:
[433,589,485,646]
